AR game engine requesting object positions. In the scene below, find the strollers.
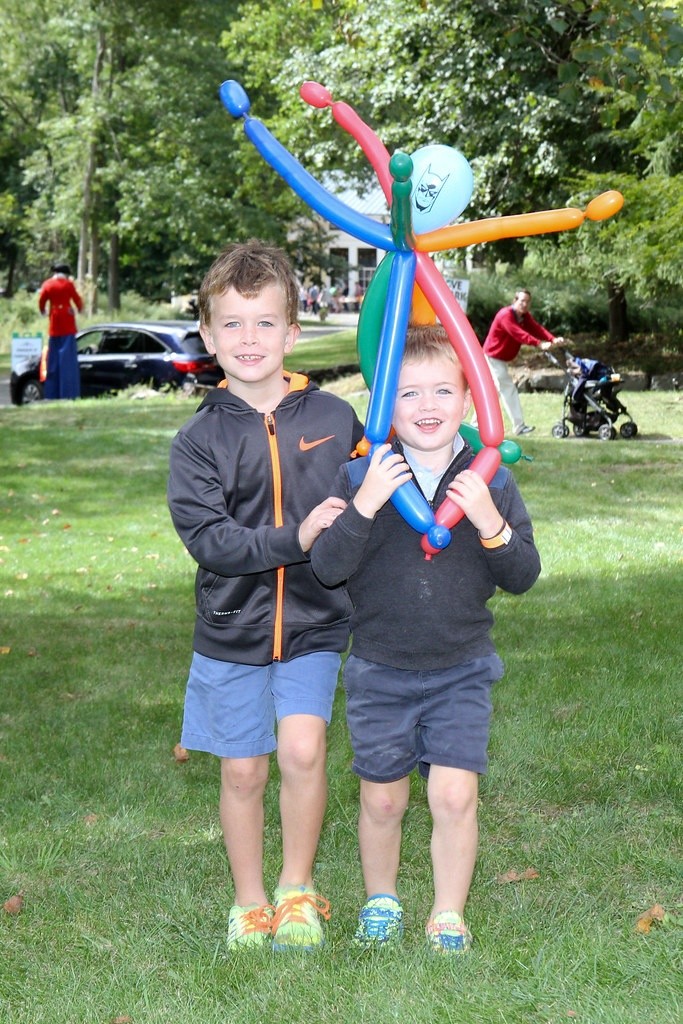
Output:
[542,340,637,440]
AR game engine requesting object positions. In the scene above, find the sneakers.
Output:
[354,894,404,950]
[425,911,473,957]
[270,885,327,953]
[226,905,272,953]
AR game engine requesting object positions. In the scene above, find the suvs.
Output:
[8,321,223,406]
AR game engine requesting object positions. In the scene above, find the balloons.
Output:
[217,78,625,560]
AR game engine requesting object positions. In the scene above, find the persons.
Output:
[306,326,541,951]
[168,239,373,951]
[39,264,88,401]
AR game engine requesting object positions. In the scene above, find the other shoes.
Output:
[518,424,536,431]
